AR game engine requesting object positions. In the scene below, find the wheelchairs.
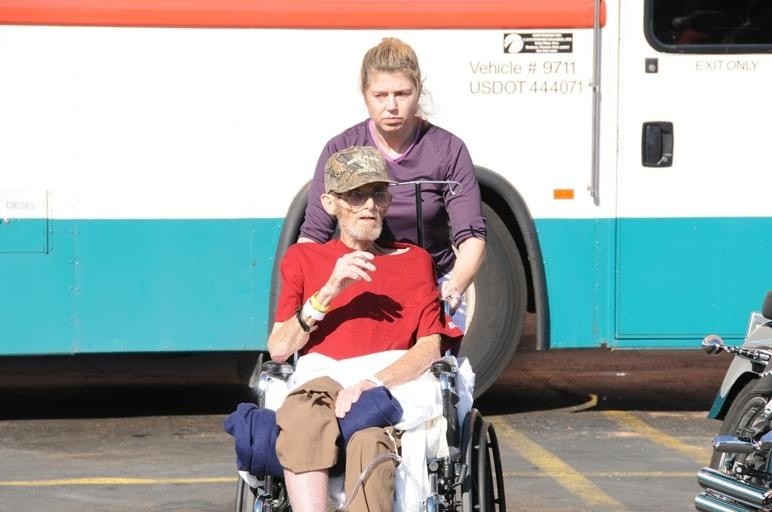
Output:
[235,179,507,512]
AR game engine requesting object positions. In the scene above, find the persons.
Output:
[298,37,488,317]
[264,145,464,512]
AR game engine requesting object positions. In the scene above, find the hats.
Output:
[324,146,399,194]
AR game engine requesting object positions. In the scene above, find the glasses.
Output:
[330,190,392,208]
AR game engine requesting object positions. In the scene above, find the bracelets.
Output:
[292,291,331,335]
[361,375,384,391]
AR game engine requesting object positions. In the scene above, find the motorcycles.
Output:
[694,289,772,512]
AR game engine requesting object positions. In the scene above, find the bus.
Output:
[1,0,772,358]
